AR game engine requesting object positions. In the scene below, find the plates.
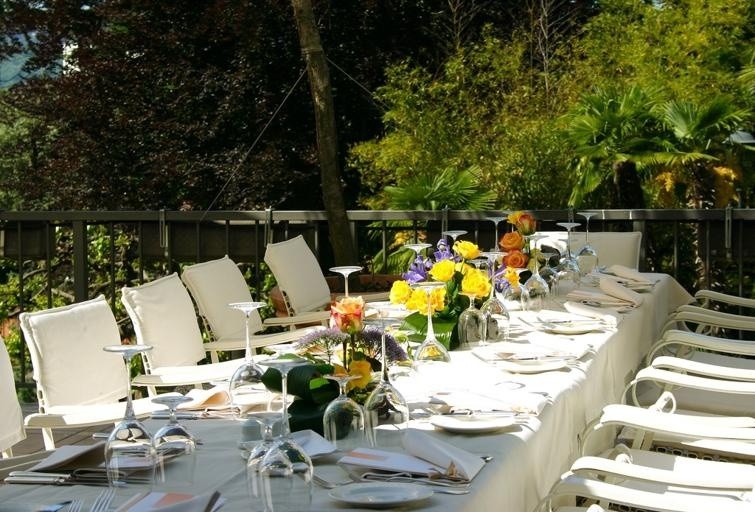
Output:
[241,427,336,464]
[431,411,517,434]
[327,481,435,505]
[582,271,659,315]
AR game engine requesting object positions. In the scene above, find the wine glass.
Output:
[229,302,269,423]
[402,210,601,395]
[360,318,409,451]
[245,389,294,503]
[102,343,196,493]
[322,373,364,453]
[260,357,314,511]
[328,267,363,332]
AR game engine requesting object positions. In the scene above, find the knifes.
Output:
[4,471,150,486]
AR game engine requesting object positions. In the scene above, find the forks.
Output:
[69,488,118,512]
[425,406,531,424]
[299,464,471,495]
